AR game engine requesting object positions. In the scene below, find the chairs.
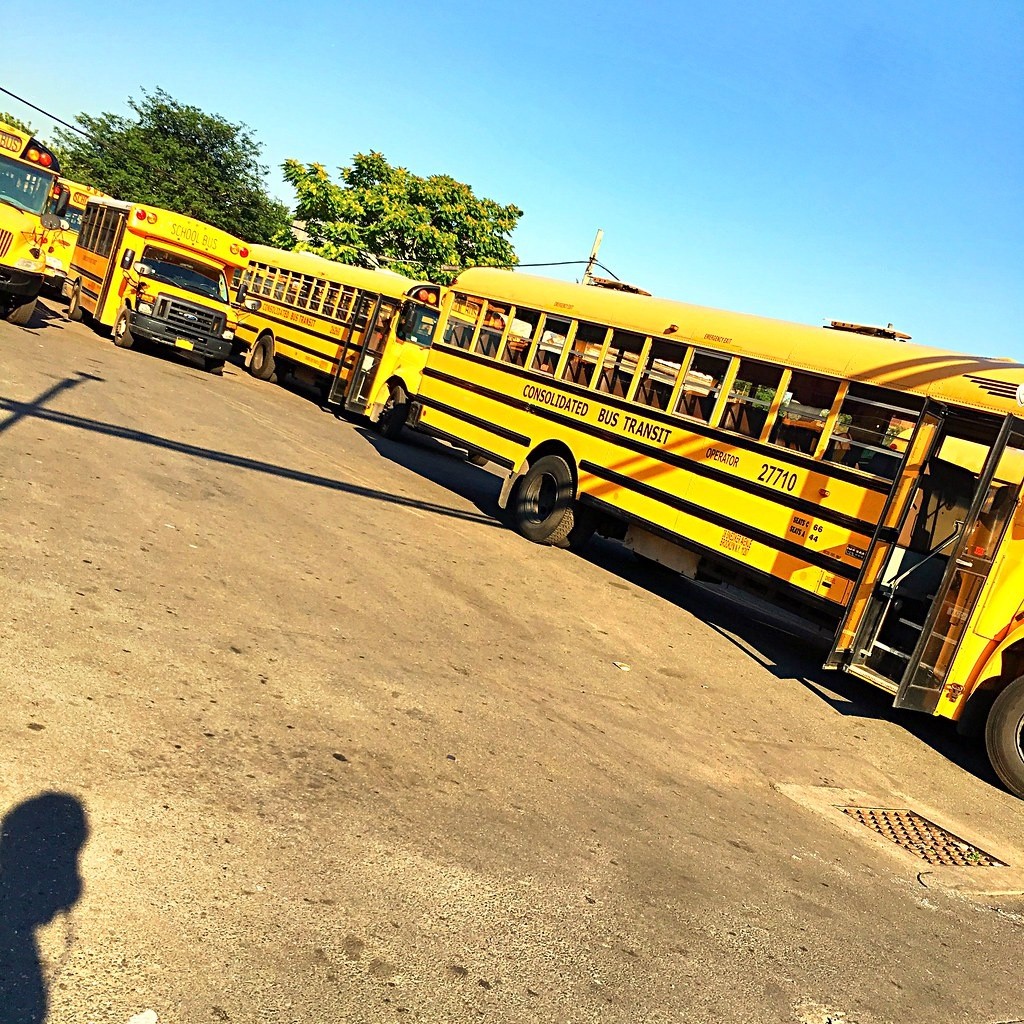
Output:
[830,434,856,466]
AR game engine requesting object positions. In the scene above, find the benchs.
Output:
[637,379,674,411]
[678,390,715,421]
[564,358,596,387]
[502,341,530,366]
[475,334,501,357]
[774,418,821,454]
[450,326,474,351]
[532,350,560,375]
[724,403,769,439]
[243,279,355,324]
[600,369,634,398]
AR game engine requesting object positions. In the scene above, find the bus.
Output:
[61,195,263,375]
[415,267,1024,803]
[888,426,1023,492]
[44,176,117,287]
[0,120,72,326]
[230,244,507,467]
